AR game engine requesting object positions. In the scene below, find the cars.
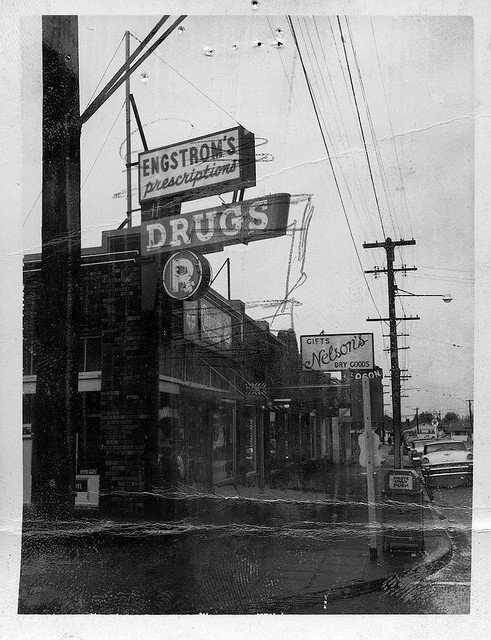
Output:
[407,438,437,468]
[420,440,472,476]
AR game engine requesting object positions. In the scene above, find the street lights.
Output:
[389,294,453,469]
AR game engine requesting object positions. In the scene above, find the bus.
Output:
[402,429,416,447]
